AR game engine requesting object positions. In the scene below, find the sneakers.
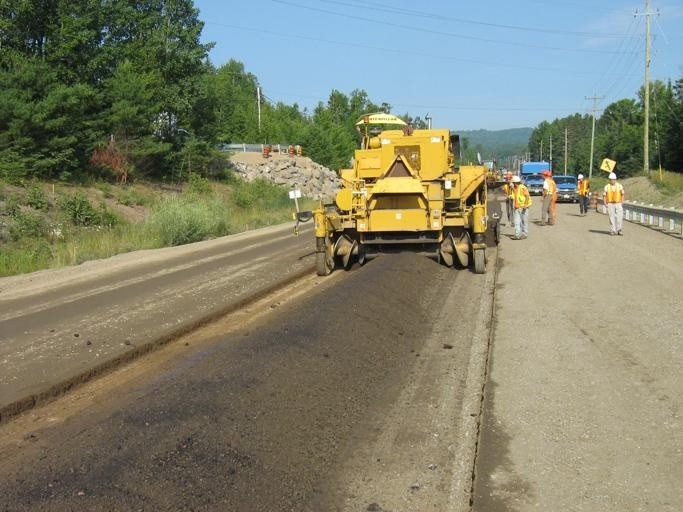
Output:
[618,229,623,236]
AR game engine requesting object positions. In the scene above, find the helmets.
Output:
[608,172,617,180]
[577,173,584,180]
[542,170,552,177]
[507,172,512,177]
[511,176,521,183]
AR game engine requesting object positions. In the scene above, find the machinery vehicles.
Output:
[283,112,504,278]
[479,158,503,190]
[497,166,509,180]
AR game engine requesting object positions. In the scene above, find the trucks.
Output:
[518,160,550,182]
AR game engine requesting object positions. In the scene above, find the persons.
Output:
[547,172,556,224]
[575,173,589,217]
[500,173,514,226]
[507,176,531,240]
[536,170,553,225]
[602,172,624,236]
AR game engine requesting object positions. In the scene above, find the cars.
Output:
[550,175,580,204]
[522,174,547,195]
